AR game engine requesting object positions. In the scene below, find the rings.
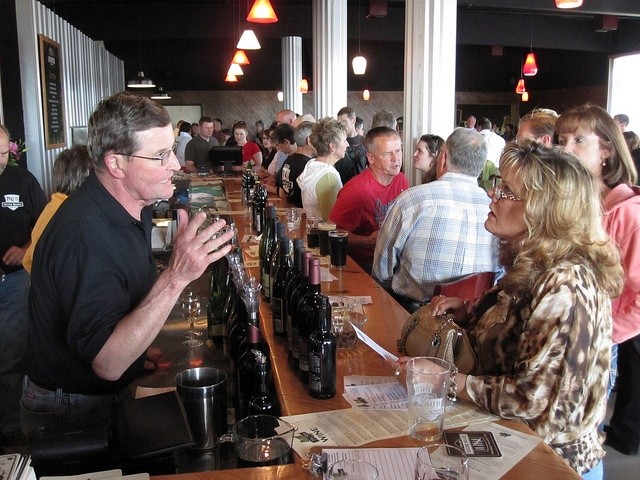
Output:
[393,365,401,377]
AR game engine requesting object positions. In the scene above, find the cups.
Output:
[319,223,336,256]
[288,208,300,230]
[176,366,226,450]
[328,231,349,271]
[329,296,367,349]
[307,216,322,249]
[415,444,469,480]
[406,357,450,442]
[323,460,379,480]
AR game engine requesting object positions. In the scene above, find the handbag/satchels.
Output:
[396,301,478,403]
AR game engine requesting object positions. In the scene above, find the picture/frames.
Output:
[38,35,68,151]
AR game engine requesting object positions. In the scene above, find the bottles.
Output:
[270,223,285,304]
[292,252,314,370]
[273,235,292,336]
[298,258,328,385]
[259,204,275,290]
[240,159,266,232]
[265,216,279,301]
[308,297,336,399]
[289,247,307,359]
[208,231,281,416]
[285,239,304,343]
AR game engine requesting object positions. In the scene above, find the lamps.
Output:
[127,39,157,89]
[151,72,172,100]
[522,12,538,76]
[236,2,262,50]
[363,78,370,102]
[231,4,250,65]
[352,5,367,75]
[246,1,278,25]
[515,78,526,95]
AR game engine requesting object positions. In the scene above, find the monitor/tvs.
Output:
[211,146,243,178]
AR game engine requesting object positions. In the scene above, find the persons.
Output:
[601,338,639,456]
[254,120,269,156]
[295,118,349,221]
[371,108,398,132]
[397,117,404,141]
[456,115,516,168]
[261,129,278,170]
[232,121,263,173]
[354,117,364,138]
[174,117,233,171]
[18,91,235,437]
[370,128,508,316]
[333,107,367,186]
[386,138,617,480]
[555,104,640,433]
[22,144,92,275]
[413,134,446,185]
[281,121,318,208]
[326,126,409,276]
[0,124,49,375]
[613,114,629,132]
[260,123,298,199]
[516,107,558,149]
[623,132,640,175]
[268,110,299,176]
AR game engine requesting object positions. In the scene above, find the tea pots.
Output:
[215,415,298,470]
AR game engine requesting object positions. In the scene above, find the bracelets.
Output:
[446,367,457,401]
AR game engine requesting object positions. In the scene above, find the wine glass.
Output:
[180,295,203,346]
[180,288,203,338]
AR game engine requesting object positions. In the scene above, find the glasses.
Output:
[487,173,526,204]
[261,137,270,140]
[113,139,181,166]
